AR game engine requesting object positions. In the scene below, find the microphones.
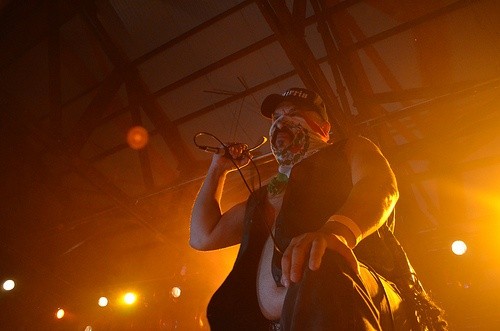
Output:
[198,144,245,162]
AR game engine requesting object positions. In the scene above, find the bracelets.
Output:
[323,213,364,248]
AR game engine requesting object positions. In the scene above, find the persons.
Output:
[187,87,451,331]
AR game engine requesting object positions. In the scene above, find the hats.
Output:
[261,87,327,123]
